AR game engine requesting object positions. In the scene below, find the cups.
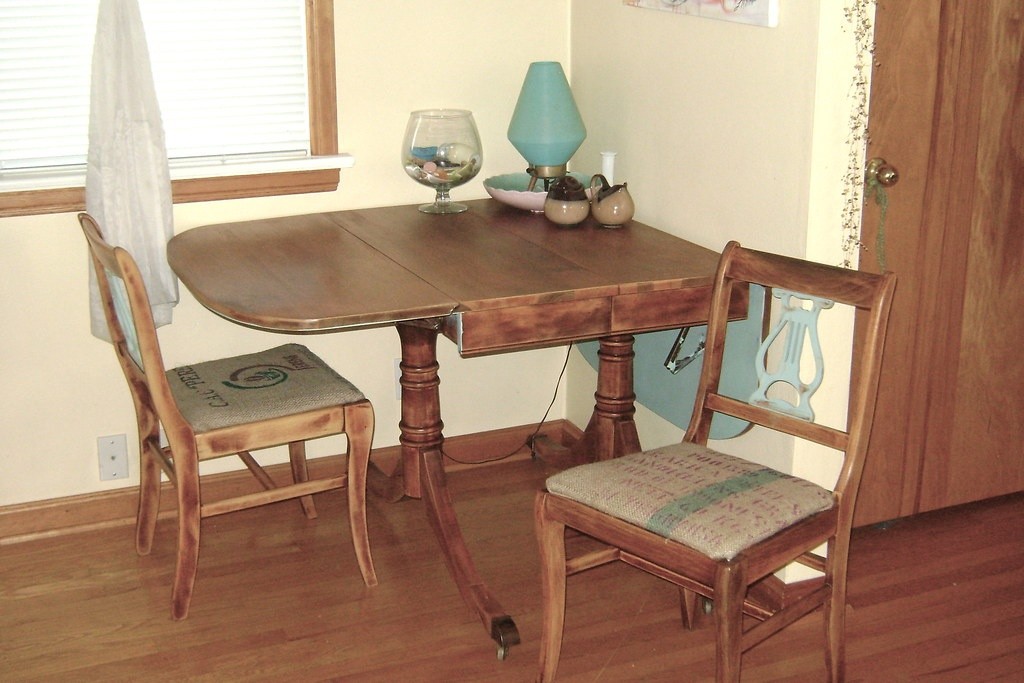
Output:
[544,174,635,229]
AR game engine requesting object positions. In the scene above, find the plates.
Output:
[483,170,603,213]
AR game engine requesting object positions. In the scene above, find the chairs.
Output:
[76,213,377,621]
[534,241,897,683]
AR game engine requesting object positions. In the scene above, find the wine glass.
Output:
[400,108,483,214]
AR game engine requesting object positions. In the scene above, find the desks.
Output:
[167,198,750,661]
